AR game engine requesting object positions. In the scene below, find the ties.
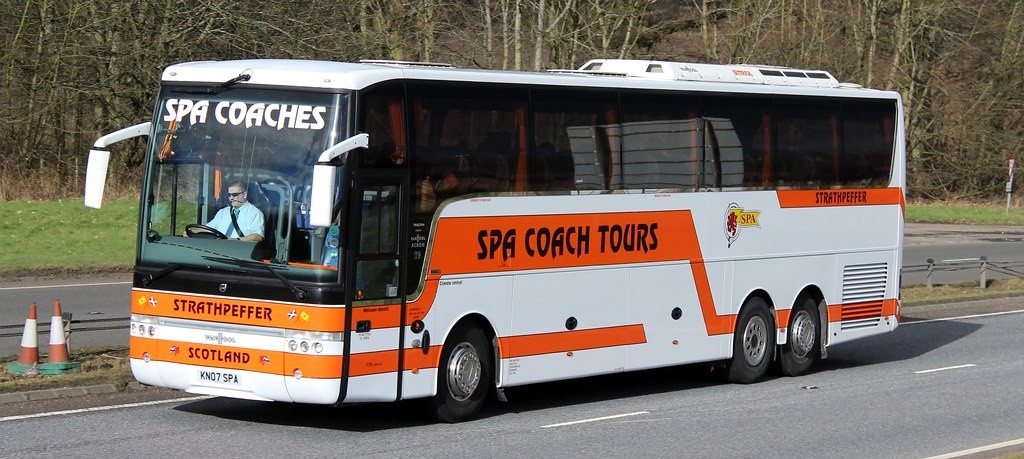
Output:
[224,209,240,239]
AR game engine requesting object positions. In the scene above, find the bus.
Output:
[85,55,908,425]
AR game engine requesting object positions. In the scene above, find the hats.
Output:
[547,139,572,157]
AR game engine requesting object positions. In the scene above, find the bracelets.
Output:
[237,237,240,241]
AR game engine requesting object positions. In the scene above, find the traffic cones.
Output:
[4,301,40,378]
[38,299,82,377]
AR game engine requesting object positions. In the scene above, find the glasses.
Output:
[227,190,245,197]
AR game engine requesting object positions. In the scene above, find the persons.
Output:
[182,181,265,242]
[431,153,488,201]
